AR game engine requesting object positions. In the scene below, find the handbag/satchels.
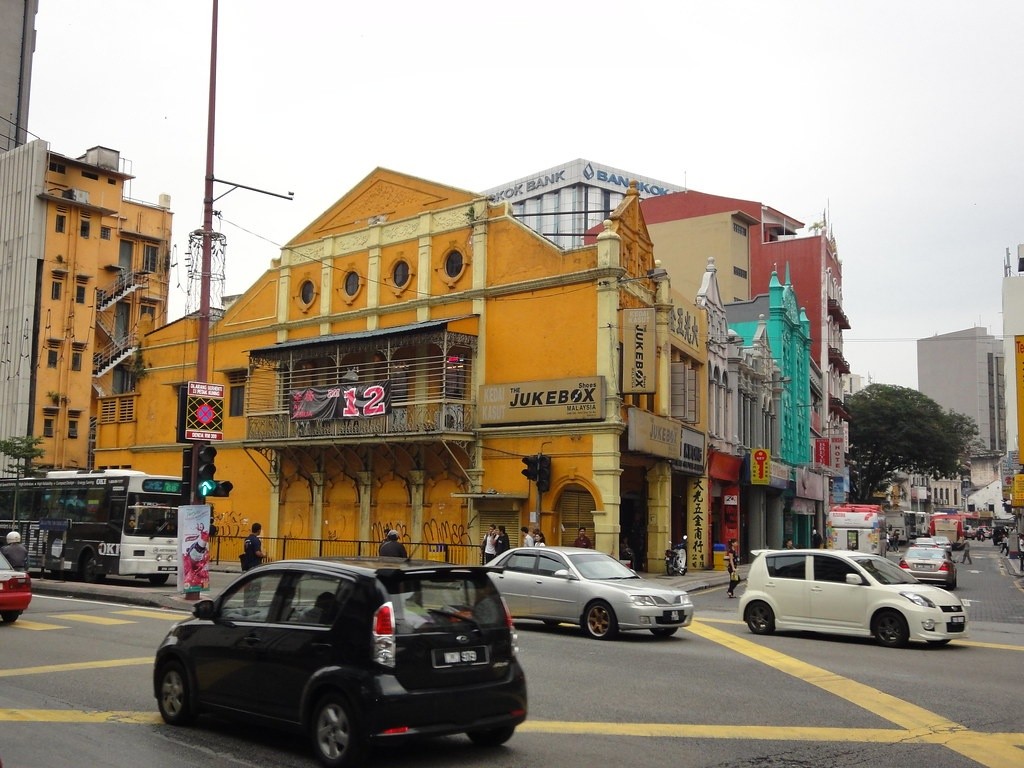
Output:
[730,571,738,581]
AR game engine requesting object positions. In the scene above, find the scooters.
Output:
[663,535,688,576]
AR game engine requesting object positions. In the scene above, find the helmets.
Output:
[388,530,399,541]
[7,532,20,544]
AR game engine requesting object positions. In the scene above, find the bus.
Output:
[0,469,182,587]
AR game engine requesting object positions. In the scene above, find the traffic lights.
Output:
[535,456,550,492]
[195,445,233,498]
[521,455,538,481]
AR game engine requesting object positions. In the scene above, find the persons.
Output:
[977,528,985,541]
[627,525,642,570]
[1000,534,1007,553]
[480,524,499,564]
[620,536,634,569]
[533,529,545,545]
[886,532,891,551]
[243,523,267,616]
[783,539,797,550]
[726,538,741,598]
[960,540,972,564]
[187,522,208,556]
[925,529,931,537]
[379,528,409,623]
[492,525,510,556]
[813,531,822,548]
[535,532,545,547]
[892,531,899,553]
[1017,533,1024,571]
[299,591,335,624]
[573,527,592,549]
[18,502,82,521]
[520,527,535,547]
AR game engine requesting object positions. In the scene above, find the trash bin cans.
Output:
[428,544,447,562]
[713,544,727,571]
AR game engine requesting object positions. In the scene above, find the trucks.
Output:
[827,504,927,559]
[929,512,969,551]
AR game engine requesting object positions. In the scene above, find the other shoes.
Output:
[727,591,736,598]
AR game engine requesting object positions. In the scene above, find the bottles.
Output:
[187,532,209,564]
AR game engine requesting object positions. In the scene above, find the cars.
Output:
[963,525,994,539]
[738,547,969,649]
[152,557,528,768]
[0,551,32,622]
[482,547,694,641]
[897,535,958,591]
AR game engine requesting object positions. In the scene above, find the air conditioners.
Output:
[436,410,463,432]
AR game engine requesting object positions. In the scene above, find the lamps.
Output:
[619,269,667,286]
[763,377,793,384]
[797,403,823,407]
[823,425,838,431]
[706,337,744,348]
